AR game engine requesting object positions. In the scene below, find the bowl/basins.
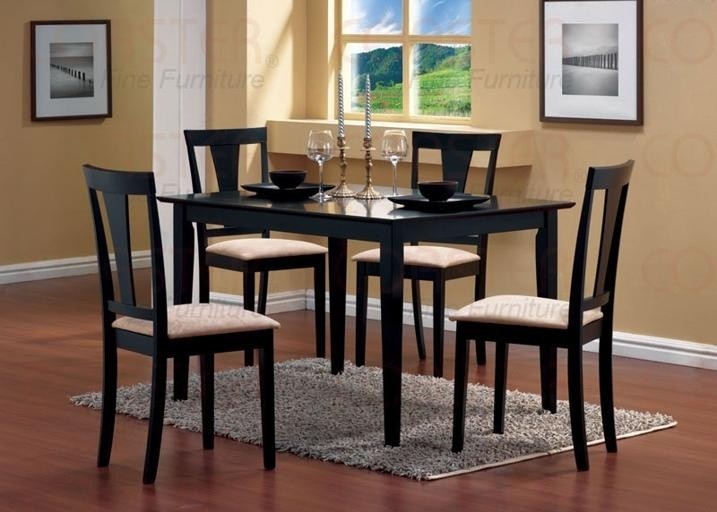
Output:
[418,181,460,202]
[269,170,307,189]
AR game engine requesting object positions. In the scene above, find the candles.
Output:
[364,72,372,140]
[336,71,345,139]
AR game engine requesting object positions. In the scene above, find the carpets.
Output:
[69,357,681,483]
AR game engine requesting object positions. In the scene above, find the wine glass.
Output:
[381,129,409,199]
[305,130,335,201]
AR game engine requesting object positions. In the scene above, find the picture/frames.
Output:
[29,19,112,121]
[537,0,644,126]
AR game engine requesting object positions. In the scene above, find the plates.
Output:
[388,194,489,209]
[239,183,336,200]
